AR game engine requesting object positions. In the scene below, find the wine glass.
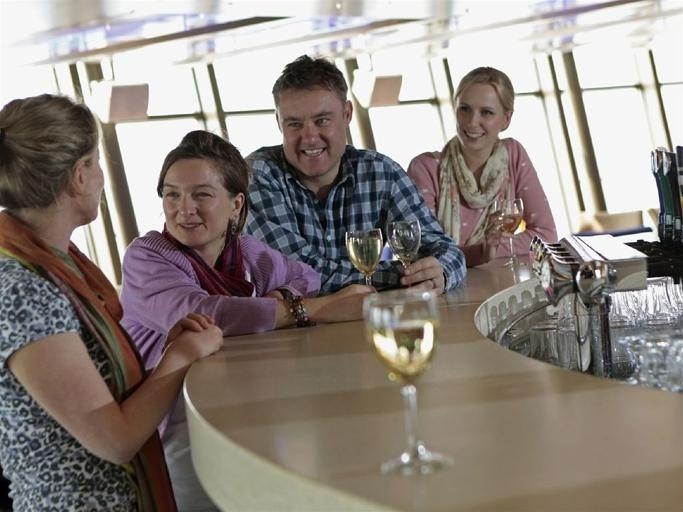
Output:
[495,199,524,271]
[361,291,454,475]
[344,221,421,287]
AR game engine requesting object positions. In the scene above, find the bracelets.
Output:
[285,293,314,327]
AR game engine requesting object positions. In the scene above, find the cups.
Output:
[526,276,682,389]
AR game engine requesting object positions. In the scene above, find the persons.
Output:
[119,131,377,512]
[407,66,558,268]
[244,54,467,297]
[0,93,224,512]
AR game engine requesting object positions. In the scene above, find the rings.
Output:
[429,278,437,289]
